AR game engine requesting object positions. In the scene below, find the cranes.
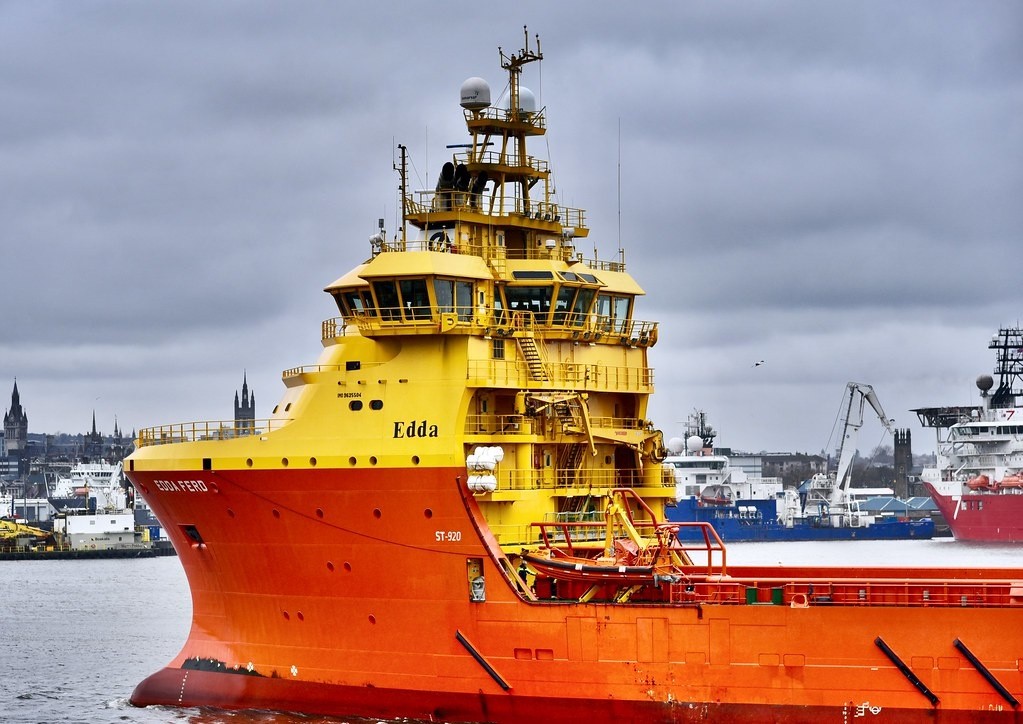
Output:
[823,381,895,491]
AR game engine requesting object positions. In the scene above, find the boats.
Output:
[0,443,177,560]
[662,408,953,542]
[964,472,1023,492]
[908,319,1023,543]
[121,23,1023,724]
